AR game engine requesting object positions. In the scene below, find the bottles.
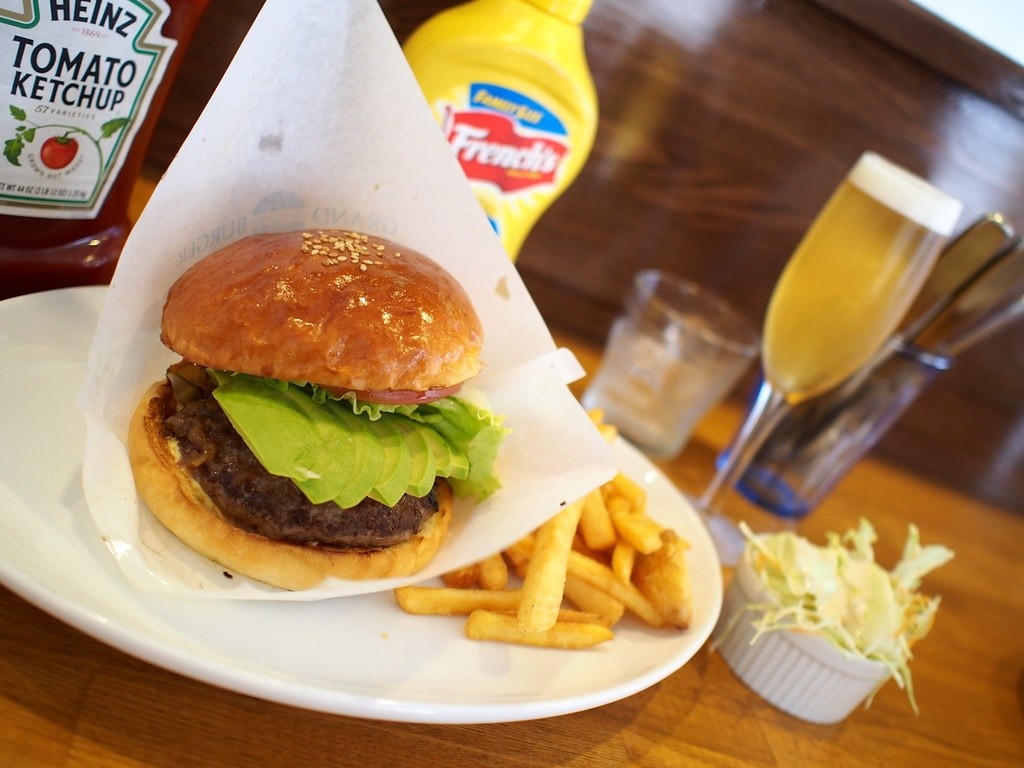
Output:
[400,1,601,269]
[0,1,208,300]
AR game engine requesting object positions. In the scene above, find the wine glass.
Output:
[682,150,963,569]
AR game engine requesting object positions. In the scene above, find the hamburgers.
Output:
[124,228,513,593]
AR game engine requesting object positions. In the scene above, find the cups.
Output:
[715,331,959,521]
[580,268,760,463]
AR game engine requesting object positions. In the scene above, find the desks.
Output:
[0,159,1024,767]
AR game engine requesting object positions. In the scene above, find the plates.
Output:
[0,286,724,726]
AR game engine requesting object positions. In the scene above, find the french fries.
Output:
[391,406,693,648]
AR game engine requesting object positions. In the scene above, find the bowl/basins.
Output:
[713,534,900,726]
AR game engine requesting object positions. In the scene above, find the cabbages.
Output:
[714,519,957,717]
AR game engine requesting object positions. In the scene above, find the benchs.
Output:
[145,0,1024,516]
